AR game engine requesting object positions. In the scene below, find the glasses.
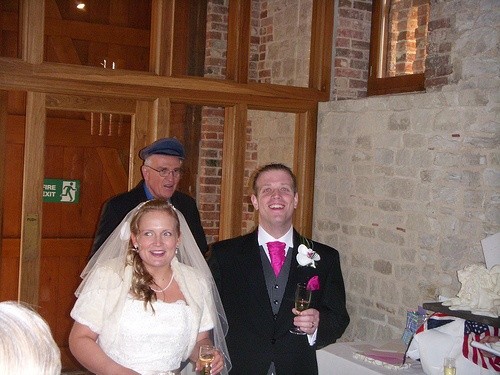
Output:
[145,165,183,177]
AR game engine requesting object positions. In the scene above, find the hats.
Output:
[139,138,185,158]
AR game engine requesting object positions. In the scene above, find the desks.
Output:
[316,340,429,375]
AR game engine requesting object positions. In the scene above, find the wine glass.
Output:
[289,282,312,336]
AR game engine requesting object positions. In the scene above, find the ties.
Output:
[266,241,286,276]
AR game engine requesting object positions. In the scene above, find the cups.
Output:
[198,345,216,375]
[444,356,456,375]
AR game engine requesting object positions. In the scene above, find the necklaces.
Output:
[151,271,174,292]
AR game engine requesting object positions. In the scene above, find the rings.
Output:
[311,322,314,328]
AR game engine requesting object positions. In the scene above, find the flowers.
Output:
[296,239,321,271]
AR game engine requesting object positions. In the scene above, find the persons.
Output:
[0,300,62,375]
[211,162,350,375]
[89,138,207,260]
[69,199,233,375]
